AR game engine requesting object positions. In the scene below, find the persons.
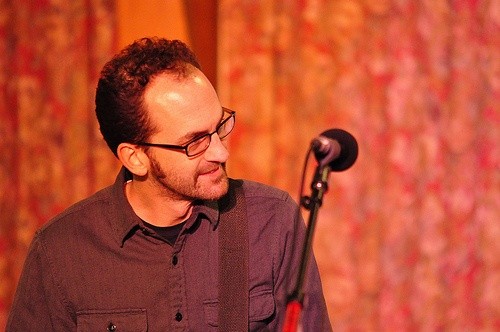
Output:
[4,35,333,332]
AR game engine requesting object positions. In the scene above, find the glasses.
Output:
[135,106,236,158]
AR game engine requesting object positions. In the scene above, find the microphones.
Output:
[309,128,360,172]
